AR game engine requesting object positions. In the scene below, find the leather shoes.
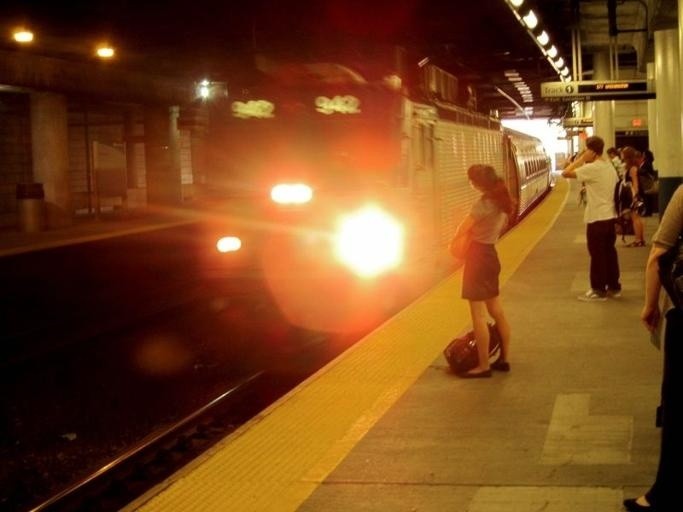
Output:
[622,493,657,510]
[492,357,508,371]
[462,367,492,379]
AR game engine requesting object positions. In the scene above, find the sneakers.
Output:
[577,287,608,302]
[609,287,623,298]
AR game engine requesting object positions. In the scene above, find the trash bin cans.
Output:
[16,182,45,230]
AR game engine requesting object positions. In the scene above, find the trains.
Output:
[176,60,554,334]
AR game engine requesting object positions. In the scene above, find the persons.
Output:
[606,144,656,249]
[449,164,517,377]
[561,136,622,302]
[622,182,683,511]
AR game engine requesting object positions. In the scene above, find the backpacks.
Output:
[615,178,635,217]
